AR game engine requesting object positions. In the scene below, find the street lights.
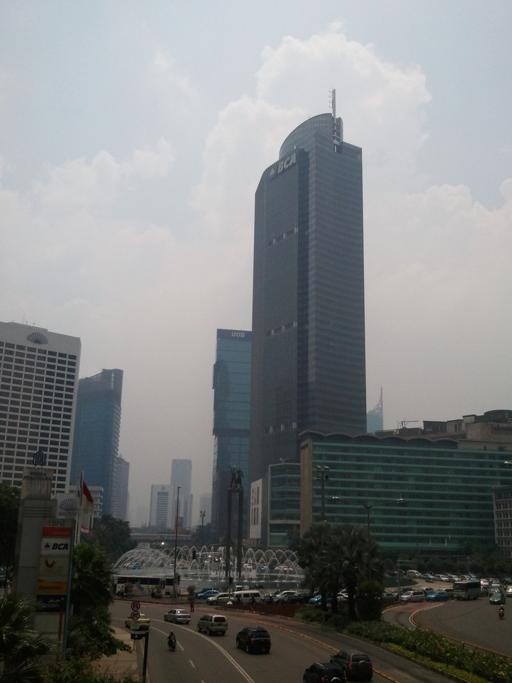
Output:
[199,510,206,527]
[332,496,406,537]
[316,465,330,553]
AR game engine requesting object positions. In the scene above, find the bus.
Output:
[112,573,181,598]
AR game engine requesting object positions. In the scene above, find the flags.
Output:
[84,480,95,503]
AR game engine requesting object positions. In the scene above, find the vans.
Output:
[199,614,228,636]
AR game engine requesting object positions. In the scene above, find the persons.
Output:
[499,605,504,610]
[169,632,176,640]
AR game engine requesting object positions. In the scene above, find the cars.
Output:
[124,614,150,631]
[236,625,271,655]
[164,609,191,625]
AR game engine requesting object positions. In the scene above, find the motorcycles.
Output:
[499,609,504,617]
[168,635,176,649]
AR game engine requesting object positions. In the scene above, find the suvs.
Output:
[304,648,372,683]
